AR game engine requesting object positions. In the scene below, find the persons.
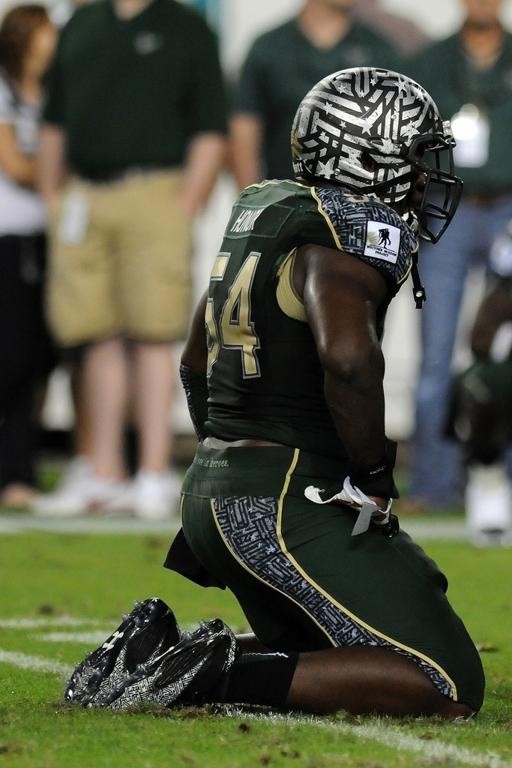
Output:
[60,62,484,714]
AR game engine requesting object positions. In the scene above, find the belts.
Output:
[463,189,512,206]
[73,163,172,183]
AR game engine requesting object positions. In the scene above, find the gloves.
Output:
[342,474,394,526]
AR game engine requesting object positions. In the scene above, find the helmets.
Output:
[290,66,455,203]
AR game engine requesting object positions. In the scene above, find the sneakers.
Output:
[463,464,511,532]
[64,598,238,710]
[32,457,179,518]
